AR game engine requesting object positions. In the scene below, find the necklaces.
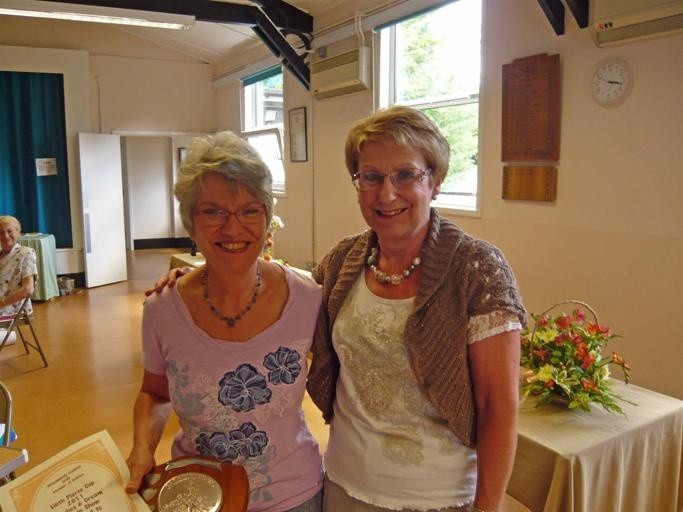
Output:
[367,238,421,284]
[197,260,262,326]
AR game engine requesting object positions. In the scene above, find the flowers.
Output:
[261,197,289,267]
[519,306,640,420]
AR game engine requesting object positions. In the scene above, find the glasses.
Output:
[351,168,432,192]
[190,207,267,227]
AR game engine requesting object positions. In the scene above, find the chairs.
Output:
[0,381,13,448]
[0,274,48,368]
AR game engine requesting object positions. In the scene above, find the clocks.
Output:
[589,56,635,110]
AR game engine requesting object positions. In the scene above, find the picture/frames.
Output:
[288,106,308,162]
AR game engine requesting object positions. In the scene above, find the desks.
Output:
[170,251,208,271]
[505,366,683,512]
[0,233,60,304]
[0,446,29,487]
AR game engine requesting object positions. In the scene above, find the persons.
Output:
[125,132,323,511]
[143,106,526,511]
[0,216,38,329]
